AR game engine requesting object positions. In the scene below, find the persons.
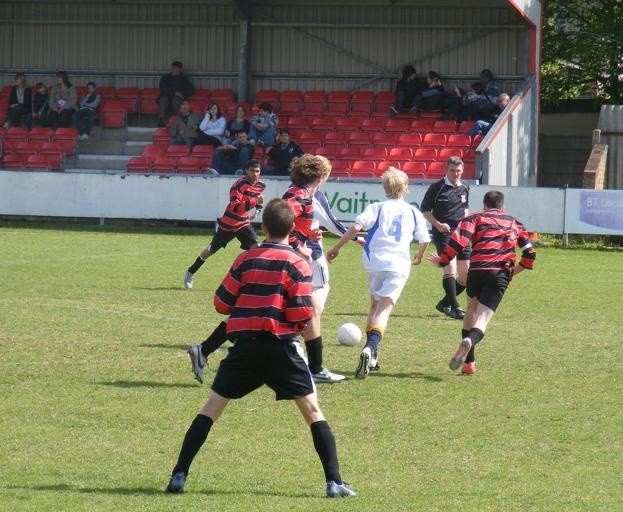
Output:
[206,129,254,174]
[183,160,266,289]
[326,167,431,379]
[424,71,444,110]
[221,105,249,147]
[46,71,77,128]
[198,102,226,147]
[426,190,536,374]
[166,198,355,497]
[75,81,100,141]
[3,73,30,132]
[420,156,472,319]
[468,93,510,136]
[462,69,497,116]
[267,130,303,174]
[247,103,279,147]
[311,155,366,315]
[156,61,193,127]
[187,153,347,383]
[28,82,48,128]
[168,101,200,149]
[389,64,421,115]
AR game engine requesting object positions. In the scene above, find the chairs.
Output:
[126,157,152,173]
[364,148,387,162]
[167,144,190,157]
[413,146,437,162]
[351,92,374,118]
[360,120,385,133]
[300,130,322,141]
[317,147,337,160]
[3,153,26,170]
[2,85,12,97]
[153,157,176,173]
[26,155,51,171]
[279,90,301,117]
[101,100,125,127]
[325,91,350,116]
[376,92,397,119]
[0,108,7,125]
[427,162,448,179]
[472,135,482,147]
[211,89,233,114]
[388,147,413,162]
[438,148,463,162]
[397,133,422,147]
[288,118,308,135]
[252,90,279,115]
[433,120,457,132]
[422,133,447,148]
[188,89,209,112]
[376,162,401,176]
[463,148,475,162]
[153,129,169,143]
[458,120,475,133]
[448,134,471,148]
[463,163,474,179]
[52,128,76,156]
[4,141,15,153]
[385,119,410,133]
[410,120,433,133]
[351,161,376,177]
[5,127,27,142]
[338,146,363,161]
[403,161,426,179]
[177,157,200,174]
[329,160,350,177]
[141,88,161,114]
[336,118,360,131]
[311,118,335,133]
[40,143,64,169]
[192,145,213,159]
[29,127,51,143]
[98,87,116,100]
[349,132,372,147]
[372,133,396,148]
[299,141,321,156]
[303,91,326,118]
[0,92,9,108]
[15,142,39,155]
[323,133,347,146]
[118,87,139,115]
[143,143,165,158]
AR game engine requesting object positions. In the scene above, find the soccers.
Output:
[336,322,362,346]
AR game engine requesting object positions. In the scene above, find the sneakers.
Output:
[183,269,194,289]
[165,470,187,493]
[434,299,451,317]
[354,347,372,377]
[368,348,380,371]
[312,367,347,384]
[389,105,399,114]
[235,168,244,175]
[449,307,466,319]
[207,167,219,175]
[186,344,209,384]
[448,336,473,371]
[408,106,419,114]
[325,479,357,498]
[461,360,476,374]
[79,133,89,140]
